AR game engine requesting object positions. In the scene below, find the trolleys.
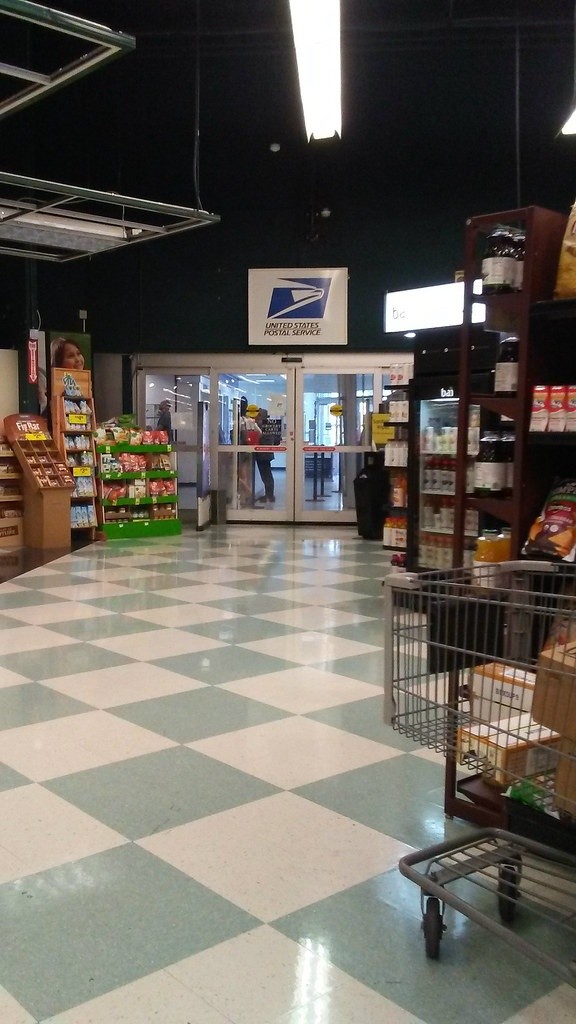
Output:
[384,558,576,999]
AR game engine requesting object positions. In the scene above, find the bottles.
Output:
[476,227,523,295]
[424,427,480,453]
[493,336,519,397]
[382,518,406,548]
[422,456,475,496]
[423,497,454,533]
[473,430,515,499]
[418,532,452,569]
[463,508,511,594]
[389,473,407,509]
[388,389,408,422]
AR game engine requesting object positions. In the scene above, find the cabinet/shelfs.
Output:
[0,450,24,547]
[50,368,98,539]
[382,321,482,614]
[442,204,576,856]
[96,442,182,541]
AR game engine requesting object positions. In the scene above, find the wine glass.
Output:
[384,441,407,468]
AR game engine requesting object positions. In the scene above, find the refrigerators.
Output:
[407,371,483,577]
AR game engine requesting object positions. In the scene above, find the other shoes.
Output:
[260,495,275,502]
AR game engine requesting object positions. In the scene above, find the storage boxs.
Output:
[458,584,576,816]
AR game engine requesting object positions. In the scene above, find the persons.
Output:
[230,399,262,505]
[254,409,275,503]
[155,400,172,441]
[51,337,85,370]
